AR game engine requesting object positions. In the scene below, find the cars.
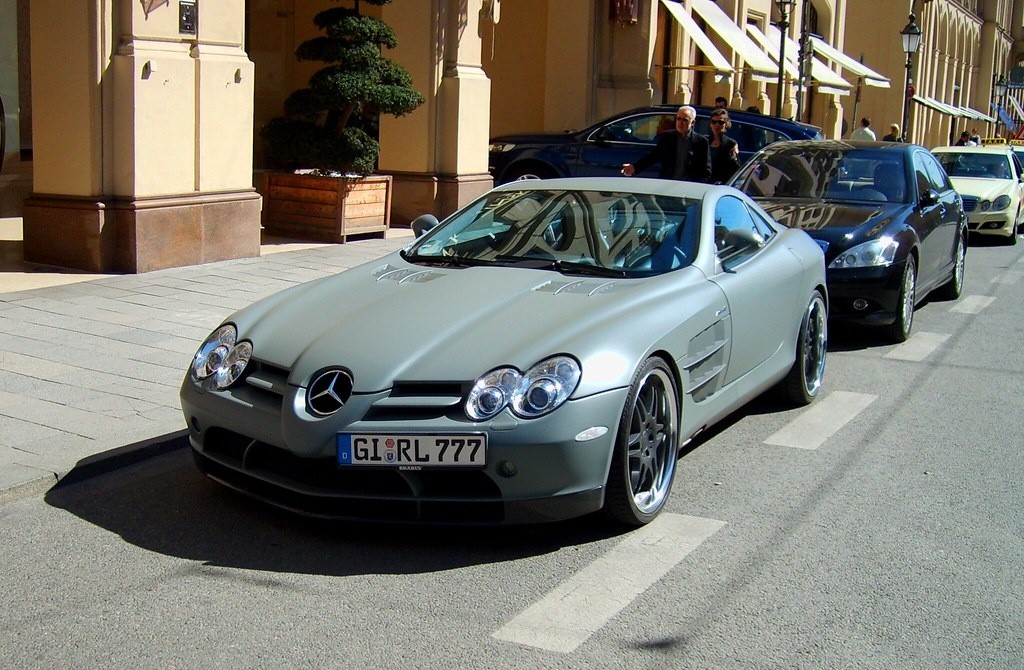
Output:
[929,145,1024,246]
[713,139,969,343]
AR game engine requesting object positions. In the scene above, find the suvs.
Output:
[487,103,824,186]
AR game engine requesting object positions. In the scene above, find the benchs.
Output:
[835,180,875,193]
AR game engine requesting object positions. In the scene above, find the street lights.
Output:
[995,74,1011,138]
[774,0,798,118]
[899,11,924,142]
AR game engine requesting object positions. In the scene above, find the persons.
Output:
[715,96,766,151]
[850,117,877,141]
[994,162,1010,178]
[882,123,903,142]
[620,106,711,183]
[704,107,739,185]
[955,128,981,146]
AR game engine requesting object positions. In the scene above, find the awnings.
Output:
[662,0,1024,132]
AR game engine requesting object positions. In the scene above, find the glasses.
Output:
[714,105,725,109]
[676,116,693,124]
[711,119,728,125]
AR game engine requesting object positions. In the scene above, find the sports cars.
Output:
[179,175,829,537]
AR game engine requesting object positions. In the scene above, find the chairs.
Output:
[948,161,960,176]
[874,163,905,203]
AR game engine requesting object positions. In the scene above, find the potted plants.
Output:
[250,1,426,245]
